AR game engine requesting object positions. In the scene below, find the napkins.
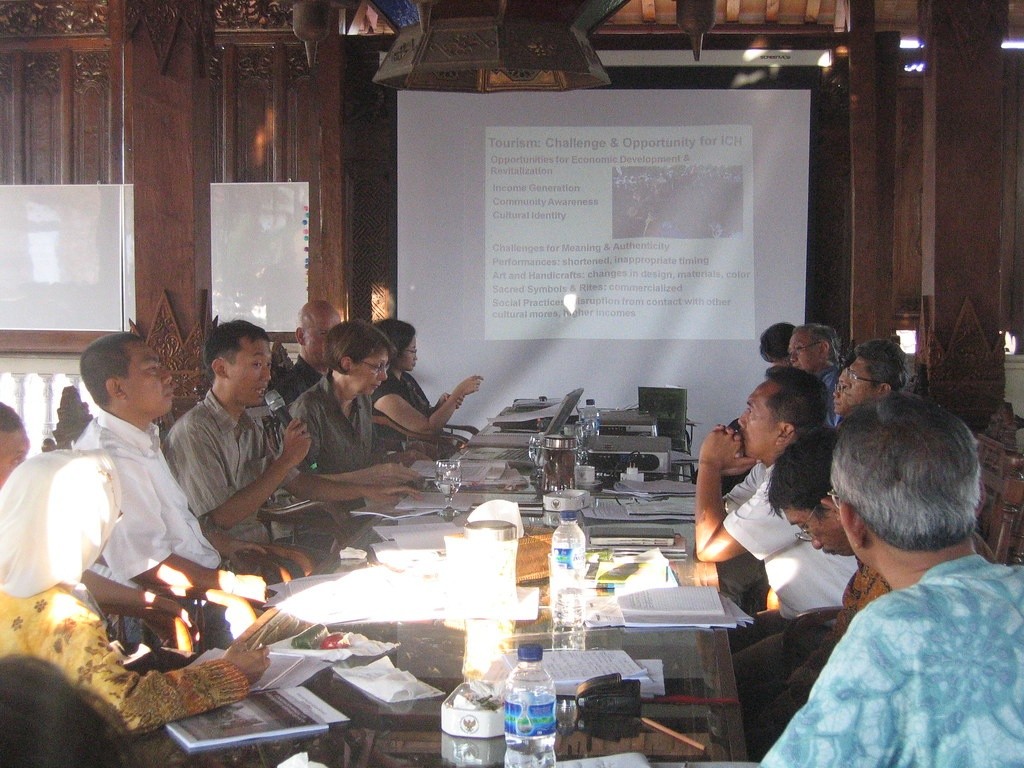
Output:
[266,632,401,661]
[332,656,446,703]
[277,752,329,768]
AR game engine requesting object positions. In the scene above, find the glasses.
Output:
[404,348,418,356]
[845,367,882,386]
[361,361,391,374]
[794,505,820,542]
[788,340,821,355]
[833,383,855,393]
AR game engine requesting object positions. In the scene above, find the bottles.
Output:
[583,398,600,436]
[550,509,586,628]
[504,643,557,768]
[545,433,576,493]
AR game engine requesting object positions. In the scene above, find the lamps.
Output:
[365,0,632,90]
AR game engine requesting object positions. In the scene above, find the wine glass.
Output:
[434,459,462,517]
[528,434,548,480]
[574,420,595,466]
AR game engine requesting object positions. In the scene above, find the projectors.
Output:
[588,434,672,474]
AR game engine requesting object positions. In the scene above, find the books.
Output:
[164,690,329,753]
[504,648,665,699]
[587,478,737,628]
[349,433,534,520]
[185,648,306,692]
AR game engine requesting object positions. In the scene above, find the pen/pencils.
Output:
[640,716,705,751]
[248,624,272,651]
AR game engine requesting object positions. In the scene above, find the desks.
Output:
[139,415,748,768]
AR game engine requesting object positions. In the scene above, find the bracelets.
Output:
[450,394,458,400]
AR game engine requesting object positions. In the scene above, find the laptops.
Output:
[457,387,584,468]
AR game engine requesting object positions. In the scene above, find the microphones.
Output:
[265,390,317,469]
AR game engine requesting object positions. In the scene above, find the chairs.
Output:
[41,339,480,654]
[782,402,1024,680]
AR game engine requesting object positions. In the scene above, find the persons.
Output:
[694,323,1024,768]
[0,301,483,768]
